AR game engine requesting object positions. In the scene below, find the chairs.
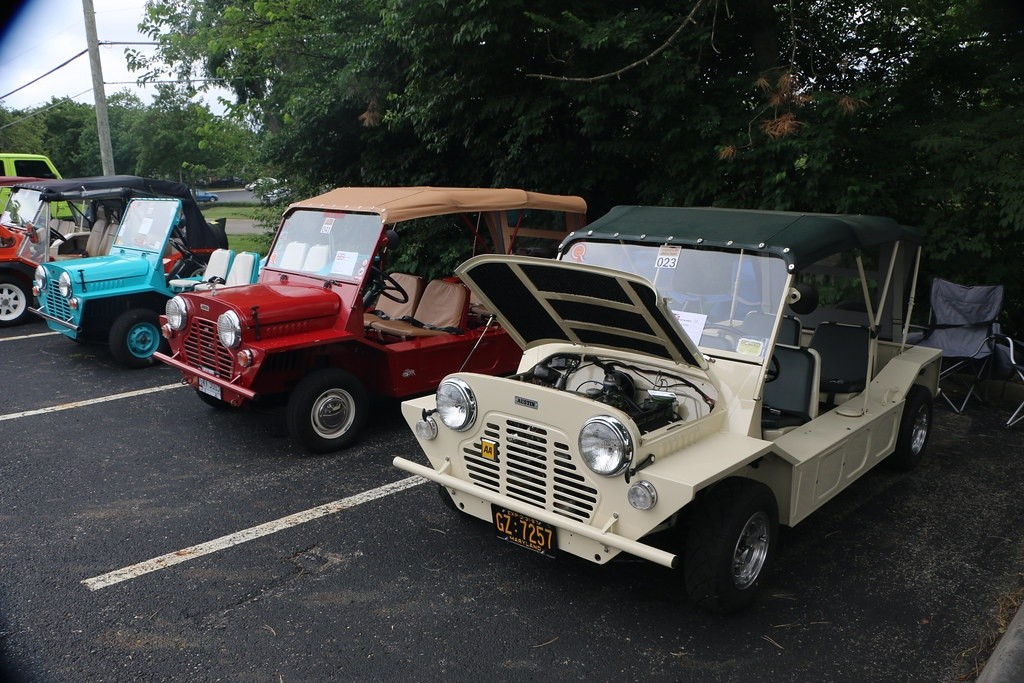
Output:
[57,219,108,261]
[737,336,821,440]
[99,222,118,256]
[808,321,874,409]
[194,252,261,291]
[469,289,497,326]
[169,248,235,293]
[363,273,423,337]
[372,277,470,344]
[744,309,802,347]
[906,277,1006,413]
[52,219,76,236]
[994,332,1024,428]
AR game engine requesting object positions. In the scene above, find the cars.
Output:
[393,205,943,596]
[0,153,371,370]
[245,177,287,192]
[153,187,587,453]
[189,189,218,202]
